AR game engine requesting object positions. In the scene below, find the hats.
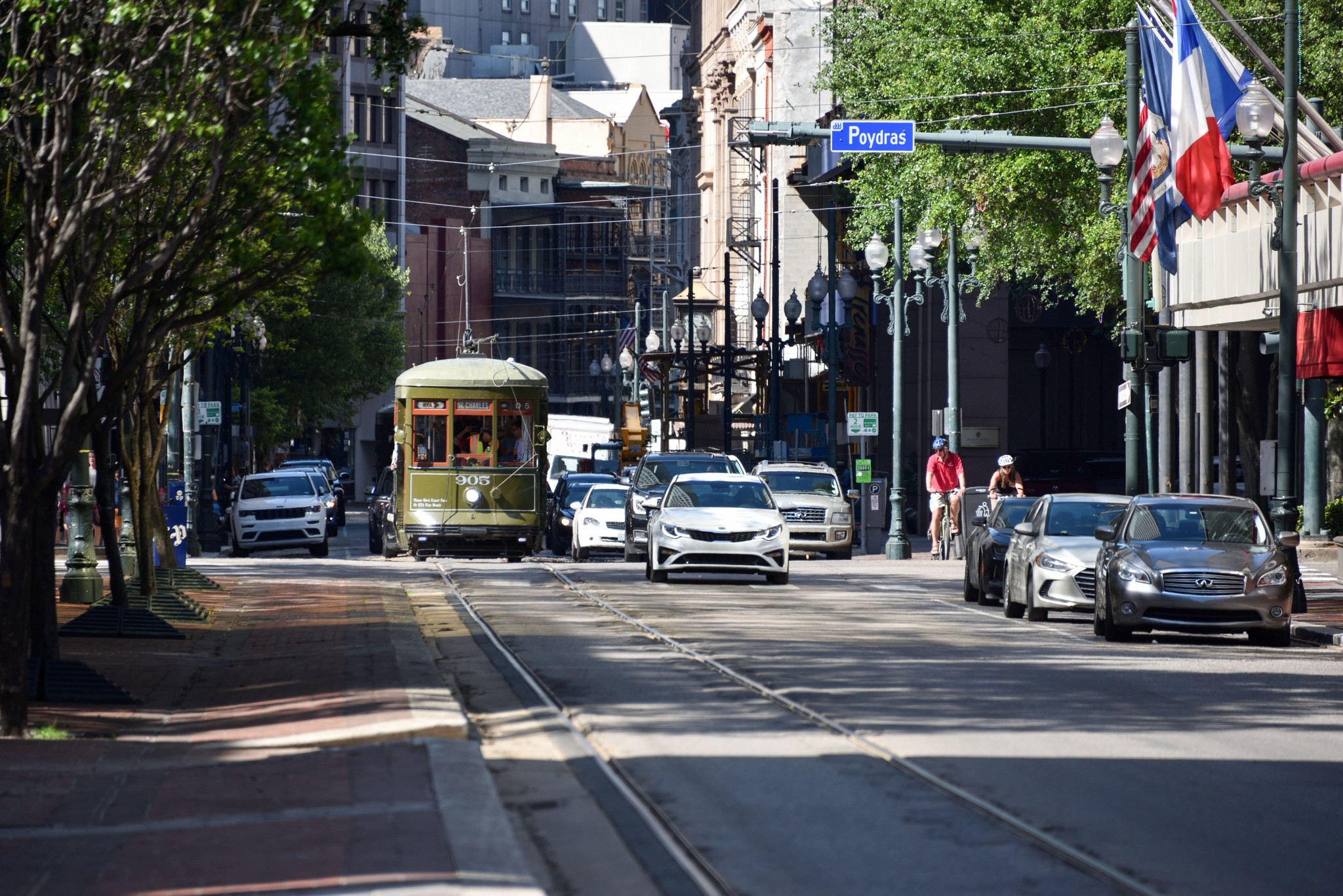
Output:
[483,428,492,432]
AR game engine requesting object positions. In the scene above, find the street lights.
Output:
[865,192,943,562]
[1087,15,1145,498]
[805,200,859,486]
[586,251,733,455]
[1232,0,1309,614]
[748,175,803,461]
[914,185,983,460]
[230,310,267,475]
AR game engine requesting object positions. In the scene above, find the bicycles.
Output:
[927,489,966,561]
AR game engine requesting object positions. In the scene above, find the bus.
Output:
[389,333,550,564]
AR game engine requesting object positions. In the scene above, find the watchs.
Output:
[961,487,966,489]
[235,486,237,488]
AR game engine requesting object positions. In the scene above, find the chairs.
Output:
[1145,511,1167,539]
[1168,519,1209,538]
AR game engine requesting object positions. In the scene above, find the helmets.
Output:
[933,438,949,448]
[998,455,1014,466]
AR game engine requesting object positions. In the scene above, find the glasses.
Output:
[511,427,518,432]
[1003,466,1012,469]
[935,448,942,452]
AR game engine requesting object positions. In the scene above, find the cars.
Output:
[1001,492,1133,623]
[1092,492,1301,648]
[640,473,799,585]
[362,464,407,558]
[226,471,336,558]
[225,456,351,557]
[962,496,1041,606]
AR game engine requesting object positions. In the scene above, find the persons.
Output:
[735,486,764,508]
[926,439,966,554]
[57,450,124,547]
[415,420,525,466]
[197,435,271,517]
[989,455,1025,511]
[703,463,732,496]
[836,461,851,498]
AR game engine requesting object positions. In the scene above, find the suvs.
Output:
[544,445,762,564]
[750,460,861,560]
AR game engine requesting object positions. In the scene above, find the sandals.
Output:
[931,545,939,554]
[951,522,958,533]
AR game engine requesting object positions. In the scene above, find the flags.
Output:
[1125,1,1275,276]
